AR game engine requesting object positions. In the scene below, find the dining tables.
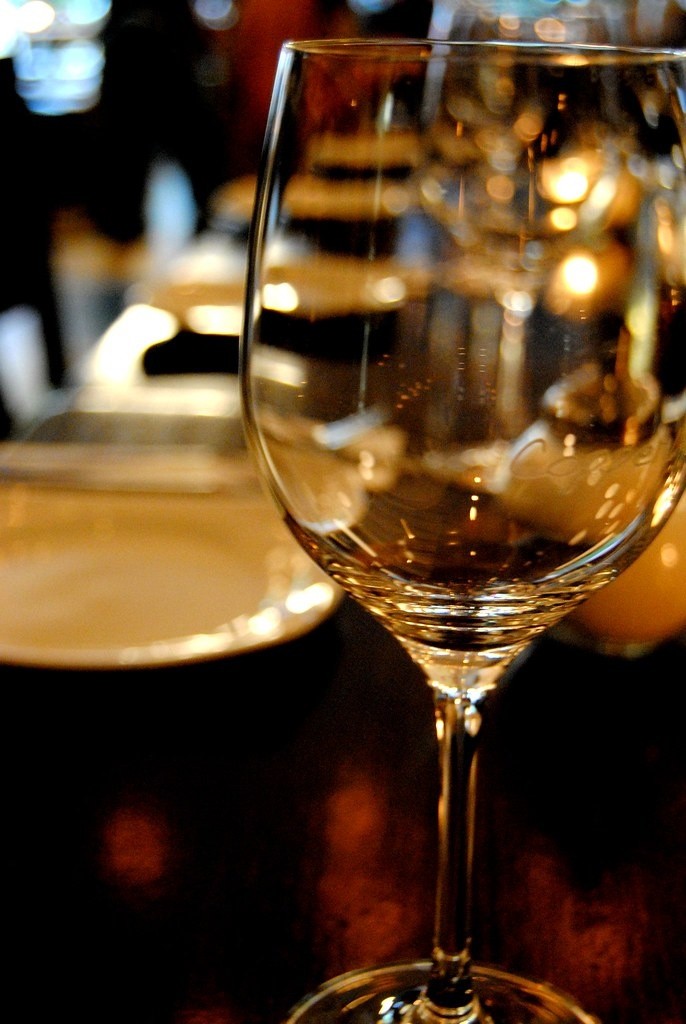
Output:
[0,168,686,1024]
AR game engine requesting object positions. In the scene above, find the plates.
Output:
[1,480,347,667]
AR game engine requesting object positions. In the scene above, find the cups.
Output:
[501,433,686,660]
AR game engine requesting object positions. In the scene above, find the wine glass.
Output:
[420,1,686,484]
[243,36,686,1024]
